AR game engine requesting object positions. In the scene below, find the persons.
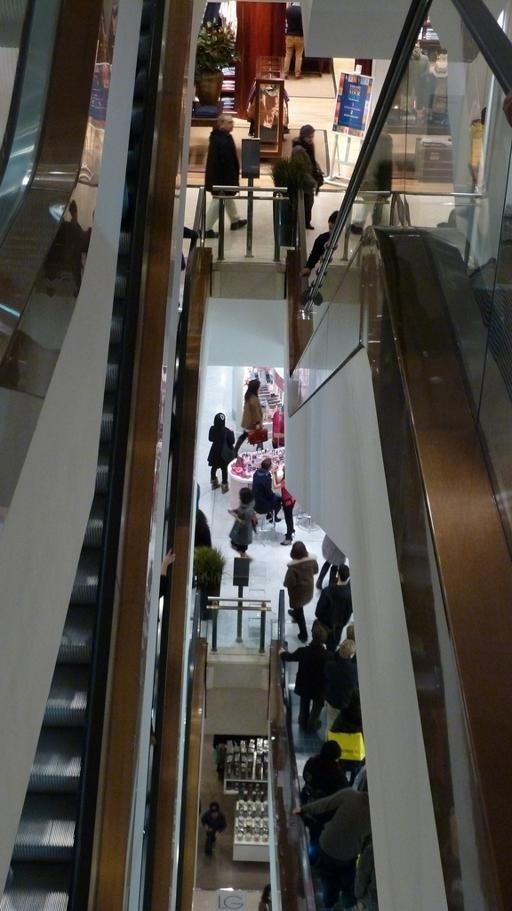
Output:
[299,210,339,278]
[270,463,297,546]
[259,883,272,911]
[347,121,394,236]
[300,282,323,309]
[200,113,248,240]
[227,487,258,564]
[40,199,90,300]
[324,710,366,792]
[315,533,346,592]
[200,801,227,864]
[292,775,371,911]
[300,739,350,806]
[216,743,225,785]
[282,540,319,643]
[324,623,360,710]
[206,413,239,495]
[177,226,199,308]
[235,379,266,456]
[279,621,330,734]
[280,1,304,79]
[314,565,353,653]
[290,124,325,231]
[161,548,178,598]
[251,459,283,523]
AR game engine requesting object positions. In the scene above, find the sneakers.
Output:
[306,224,314,229]
[288,609,297,620]
[297,634,307,643]
[205,229,218,238]
[350,224,362,234]
[230,219,247,231]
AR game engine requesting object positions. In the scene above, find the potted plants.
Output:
[191,545,223,620]
[190,11,239,110]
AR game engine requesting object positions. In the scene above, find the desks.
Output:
[188,101,224,139]
[226,448,304,525]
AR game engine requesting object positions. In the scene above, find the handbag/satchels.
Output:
[250,512,258,532]
[247,428,268,444]
[299,791,308,806]
[327,729,366,761]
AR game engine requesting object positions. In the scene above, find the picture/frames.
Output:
[254,76,285,158]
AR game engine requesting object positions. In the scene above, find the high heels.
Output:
[280,537,292,545]
[285,529,295,536]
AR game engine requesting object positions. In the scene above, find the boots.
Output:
[221,482,229,494]
[211,477,220,490]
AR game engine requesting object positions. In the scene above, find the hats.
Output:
[339,563,350,577]
[299,125,315,139]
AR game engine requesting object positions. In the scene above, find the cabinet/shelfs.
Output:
[217,737,272,862]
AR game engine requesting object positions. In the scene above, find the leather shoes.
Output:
[266,512,272,519]
[272,517,281,522]
